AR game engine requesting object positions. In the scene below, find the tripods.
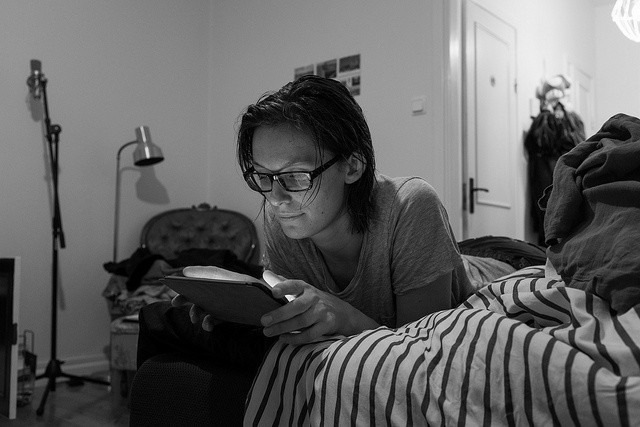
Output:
[18,80,111,417]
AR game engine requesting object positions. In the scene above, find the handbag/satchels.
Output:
[526,111,553,156]
[552,102,585,158]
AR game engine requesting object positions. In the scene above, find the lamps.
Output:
[112,126,166,262]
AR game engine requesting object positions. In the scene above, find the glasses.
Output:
[244,146,349,192]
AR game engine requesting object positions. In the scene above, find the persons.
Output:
[170,74,548,348]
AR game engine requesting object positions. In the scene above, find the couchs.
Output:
[107,202,258,384]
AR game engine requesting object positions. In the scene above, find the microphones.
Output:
[31,59,41,98]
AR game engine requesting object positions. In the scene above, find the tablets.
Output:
[164,276,290,326]
